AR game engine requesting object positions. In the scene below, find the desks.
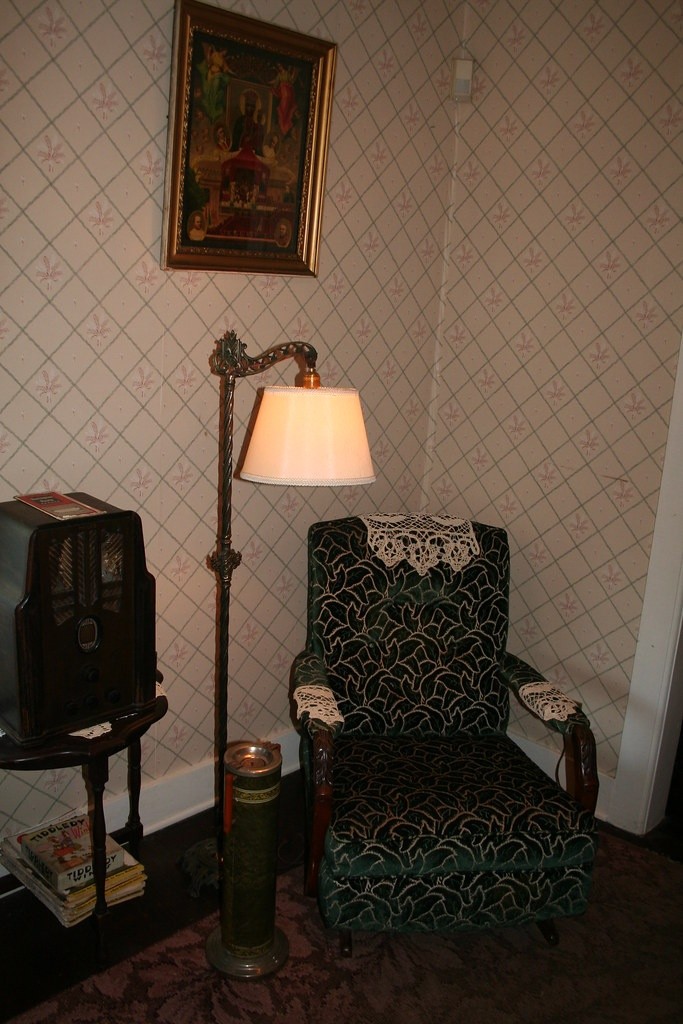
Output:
[0,679,168,923]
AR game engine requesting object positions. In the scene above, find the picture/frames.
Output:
[160,1,340,277]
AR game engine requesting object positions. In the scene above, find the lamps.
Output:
[210,330,377,891]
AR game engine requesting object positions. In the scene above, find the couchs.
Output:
[290,514,599,956]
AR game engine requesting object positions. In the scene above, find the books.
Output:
[0,807,147,927]
